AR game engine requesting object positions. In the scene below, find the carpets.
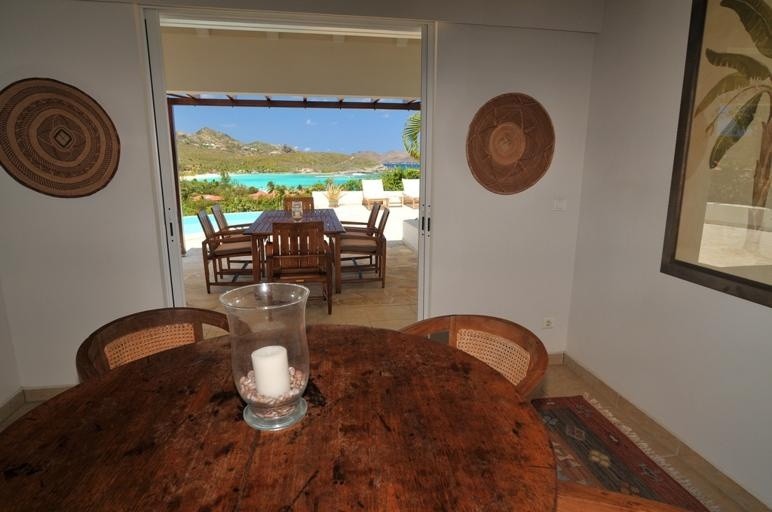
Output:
[530,392,721,512]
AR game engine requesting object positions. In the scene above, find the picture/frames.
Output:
[660,0,772,309]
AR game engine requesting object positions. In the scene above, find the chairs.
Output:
[556,479,692,512]
[398,313,549,398]
[361,179,389,210]
[75,306,253,380]
[196,197,389,323]
[402,178,420,209]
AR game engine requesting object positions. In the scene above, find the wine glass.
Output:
[217,282,313,432]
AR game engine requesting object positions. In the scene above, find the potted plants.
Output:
[323,183,345,208]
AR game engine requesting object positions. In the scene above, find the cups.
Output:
[291,201,304,223]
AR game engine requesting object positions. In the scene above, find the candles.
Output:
[250,345,290,397]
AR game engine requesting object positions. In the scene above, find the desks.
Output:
[1,322,558,512]
[385,191,404,207]
